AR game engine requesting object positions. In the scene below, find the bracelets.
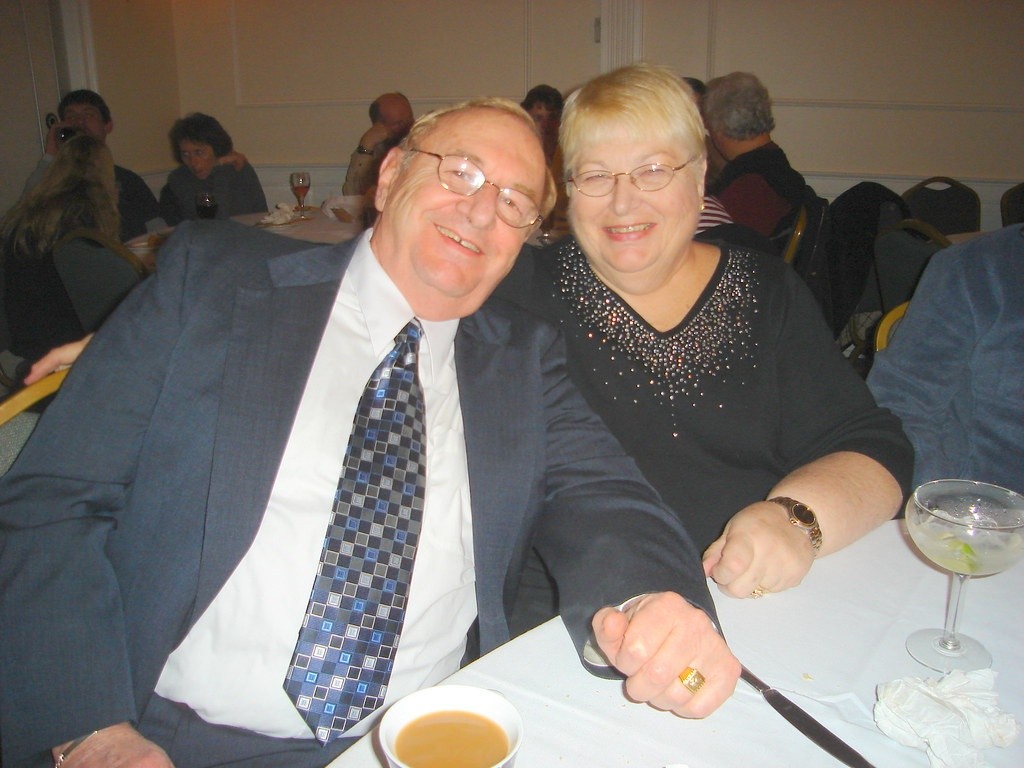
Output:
[356,145,374,155]
[54,731,98,768]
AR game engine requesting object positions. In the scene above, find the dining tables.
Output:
[323,517,1024,768]
[128,207,364,270]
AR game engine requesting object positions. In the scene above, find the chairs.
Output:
[780,176,1024,352]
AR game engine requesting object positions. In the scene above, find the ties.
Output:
[281,317,425,749]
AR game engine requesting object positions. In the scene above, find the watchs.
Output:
[767,496,823,559]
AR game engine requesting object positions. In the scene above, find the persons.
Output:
[678,72,805,258]
[340,90,414,197]
[25,63,915,598]
[864,222,1024,521]
[516,84,565,169]
[0,88,269,351]
[0,93,740,768]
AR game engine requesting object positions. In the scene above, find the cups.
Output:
[197,193,218,221]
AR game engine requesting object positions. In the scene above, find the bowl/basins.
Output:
[378,684,523,768]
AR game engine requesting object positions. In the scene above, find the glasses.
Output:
[566,156,697,196]
[403,145,544,231]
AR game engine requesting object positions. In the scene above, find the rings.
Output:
[678,667,707,695]
[752,586,766,598]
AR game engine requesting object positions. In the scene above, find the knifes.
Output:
[741,667,876,768]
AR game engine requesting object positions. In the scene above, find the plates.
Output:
[125,241,156,250]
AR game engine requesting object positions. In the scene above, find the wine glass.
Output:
[290,172,310,220]
[904,477,1024,672]
